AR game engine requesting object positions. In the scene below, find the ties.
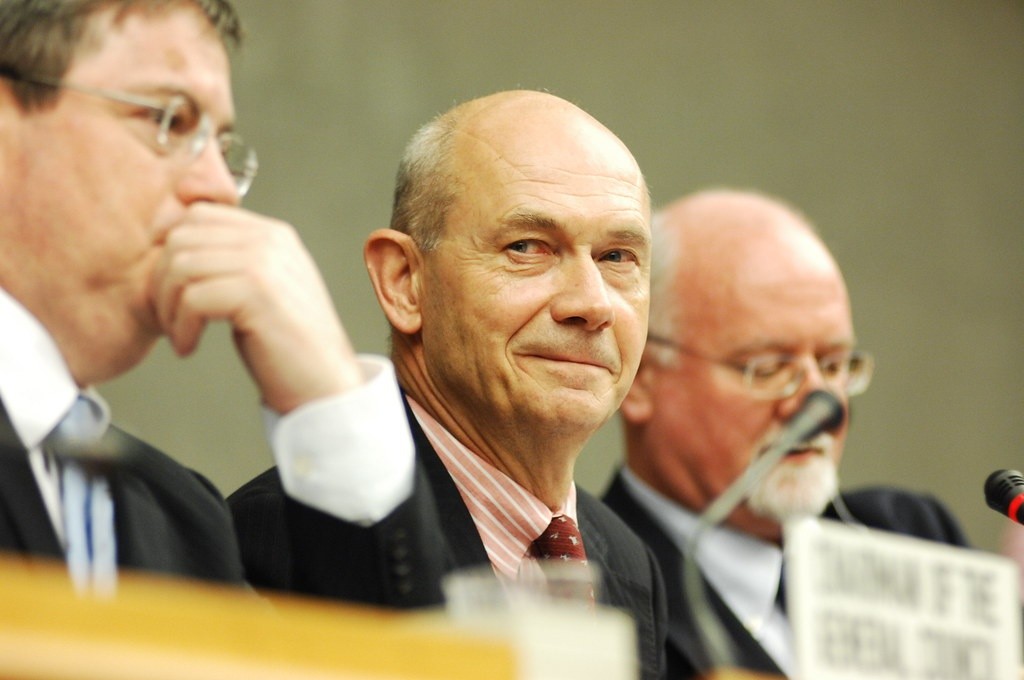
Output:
[527,515,595,622]
[54,394,117,575]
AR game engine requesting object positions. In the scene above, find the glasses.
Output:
[646,335,875,402]
[0,65,258,204]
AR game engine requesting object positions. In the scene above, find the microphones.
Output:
[984,470,1024,526]
[682,390,847,673]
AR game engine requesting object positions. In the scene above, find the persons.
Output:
[607,191,977,680]
[1,0,447,618]
[219,88,672,680]
